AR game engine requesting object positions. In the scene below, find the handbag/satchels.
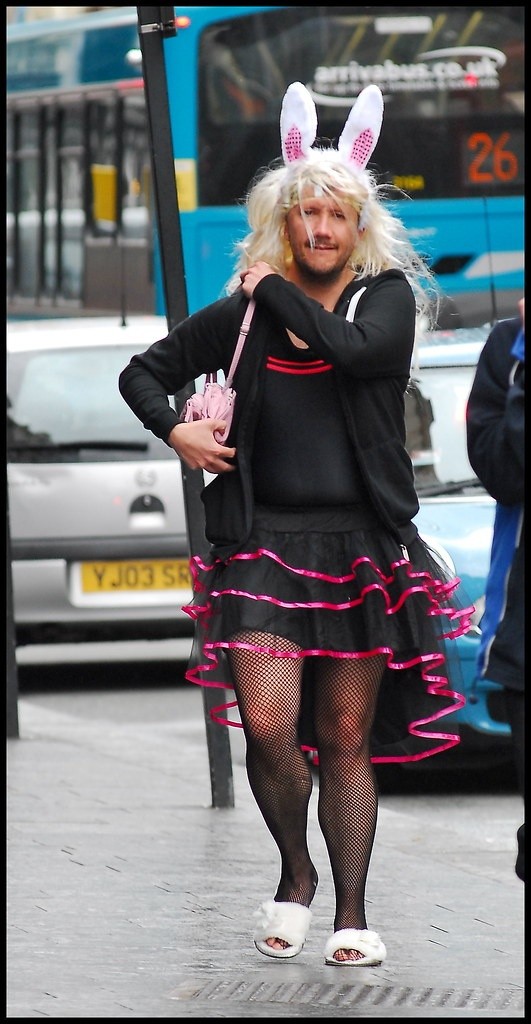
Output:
[178,296,256,446]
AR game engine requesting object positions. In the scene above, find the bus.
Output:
[6,0,530,327]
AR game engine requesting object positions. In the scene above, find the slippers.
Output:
[324,925,389,967]
[251,888,311,959]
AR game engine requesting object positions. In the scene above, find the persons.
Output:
[466,297,526,886]
[119,81,475,967]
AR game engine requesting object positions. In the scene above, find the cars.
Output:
[5,315,208,667]
[401,323,531,747]
